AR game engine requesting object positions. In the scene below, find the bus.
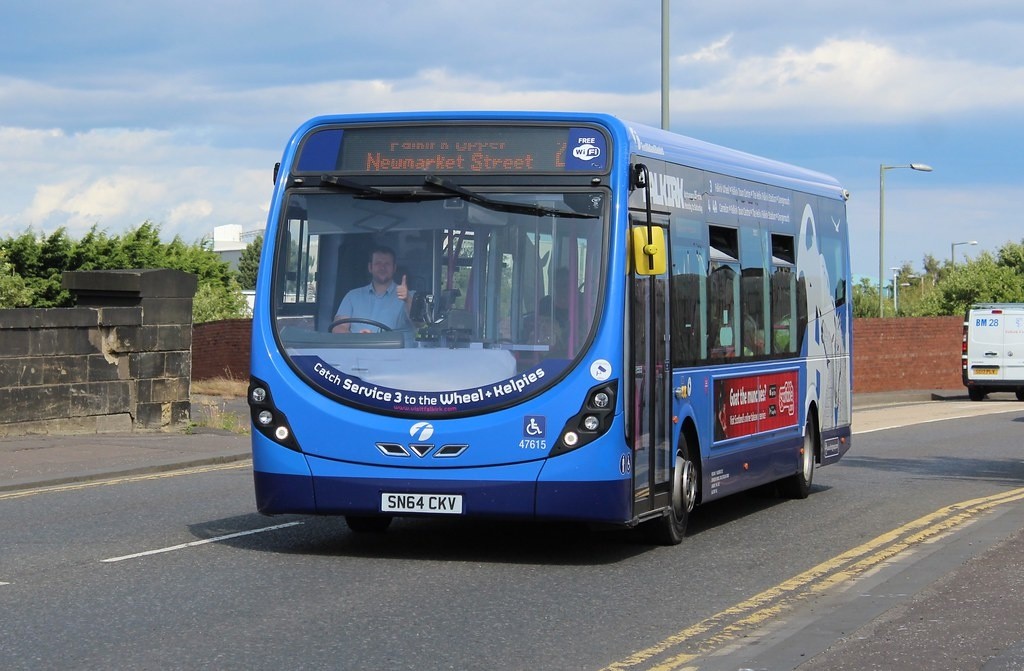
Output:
[244,110,855,550]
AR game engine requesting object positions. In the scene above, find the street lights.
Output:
[950,240,978,274]
[908,274,924,298]
[875,162,933,319]
[891,267,903,312]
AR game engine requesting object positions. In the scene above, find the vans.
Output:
[960,302,1024,403]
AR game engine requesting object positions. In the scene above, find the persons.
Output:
[331,246,419,335]
[519,266,801,367]
